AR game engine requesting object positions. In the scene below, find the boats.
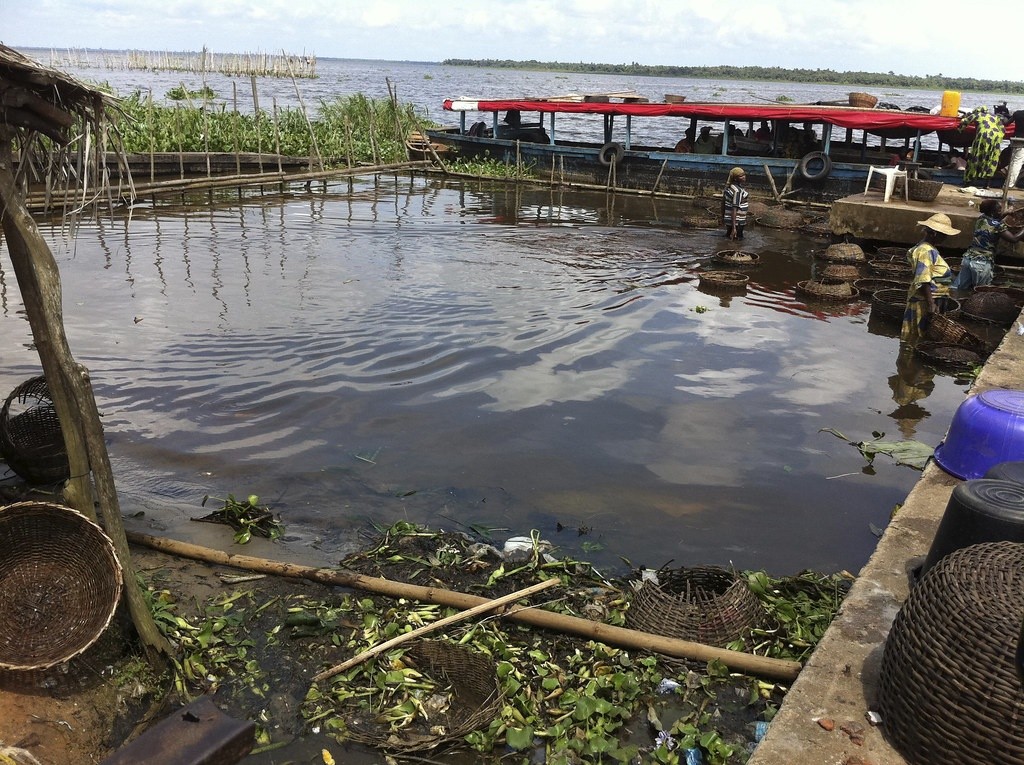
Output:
[404,124,457,163]
[422,89,1021,211]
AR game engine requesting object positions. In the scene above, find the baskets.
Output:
[702,270,749,290]
[874,246,909,262]
[931,296,961,321]
[1003,207,1024,242]
[872,289,908,321]
[341,637,506,752]
[971,285,1024,304]
[624,561,770,681]
[854,278,914,298]
[816,264,861,283]
[849,93,878,108]
[797,277,861,305]
[923,311,1008,352]
[961,290,1018,324]
[944,255,964,273]
[868,259,914,279]
[680,196,805,228]
[0,500,124,674]
[815,242,871,263]
[902,178,943,201]
[715,248,760,266]
[665,94,686,103]
[874,539,1024,765]
[916,341,989,373]
[0,373,91,481]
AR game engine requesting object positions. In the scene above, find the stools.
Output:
[865,165,908,204]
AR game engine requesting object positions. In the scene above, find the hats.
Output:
[917,213,961,236]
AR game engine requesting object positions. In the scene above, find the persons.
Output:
[900,214,961,337]
[718,121,818,155]
[694,126,717,154]
[675,128,693,153]
[887,340,936,433]
[959,107,1005,189]
[722,167,748,239]
[889,146,907,165]
[954,200,1024,289]
[906,140,921,159]
[938,150,966,170]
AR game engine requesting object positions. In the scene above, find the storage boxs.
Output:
[941,91,961,118]
[665,94,684,104]
[681,195,801,229]
[876,540,1023,764]
[0,502,121,671]
[907,178,943,202]
[718,250,759,266]
[849,91,877,108]
[701,271,748,291]
[797,242,1019,372]
